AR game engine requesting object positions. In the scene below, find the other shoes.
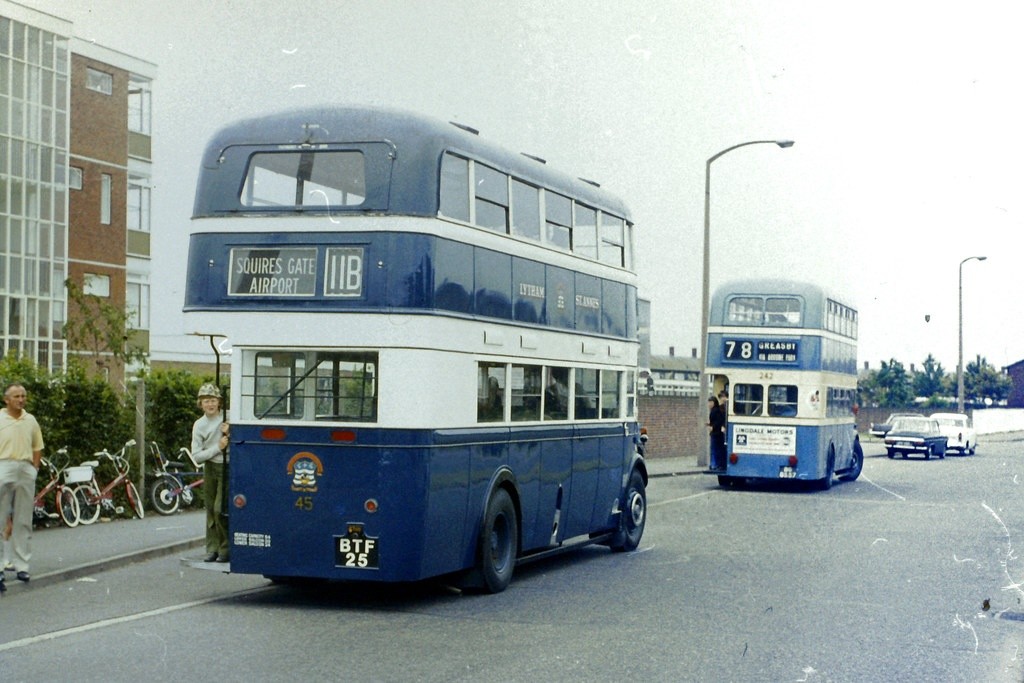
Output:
[17,571,30,581]
[216,555,230,562]
[4,562,14,570]
[204,552,219,562]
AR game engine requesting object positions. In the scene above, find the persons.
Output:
[0,384,45,591]
[708,391,728,470]
[191,384,229,562]
[705,396,727,470]
[546,369,587,413]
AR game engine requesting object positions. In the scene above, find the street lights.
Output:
[958,256,988,414]
[696,138,796,470]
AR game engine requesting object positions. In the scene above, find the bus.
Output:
[181,100,648,598]
[706,279,864,489]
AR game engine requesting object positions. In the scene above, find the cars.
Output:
[883,416,948,460]
[929,412,976,455]
[869,412,925,439]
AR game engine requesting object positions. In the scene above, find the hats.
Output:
[197,384,221,397]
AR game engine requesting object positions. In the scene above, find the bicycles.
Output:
[147,441,208,515]
[70,439,146,525]
[3,446,82,529]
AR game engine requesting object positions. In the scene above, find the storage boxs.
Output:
[63,466,93,484]
